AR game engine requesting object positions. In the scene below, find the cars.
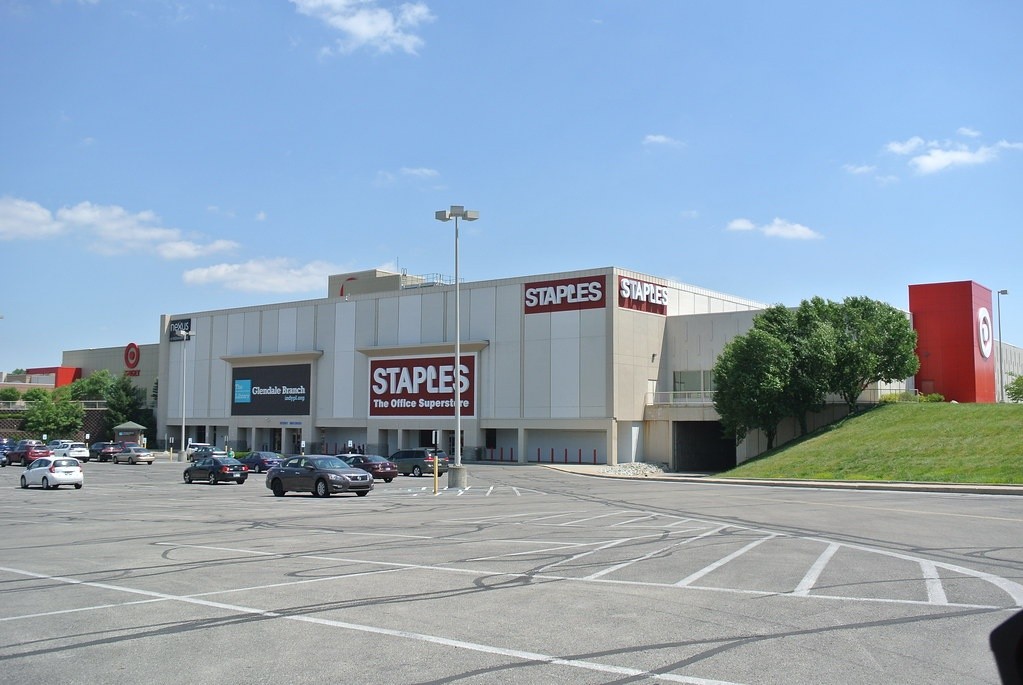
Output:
[0,437,18,467]
[89,442,123,463]
[183,456,249,485]
[189,446,228,462]
[120,442,141,450]
[20,456,83,490]
[344,455,399,483]
[237,451,285,474]
[52,442,90,463]
[111,447,156,465]
[266,454,375,498]
[333,454,362,463]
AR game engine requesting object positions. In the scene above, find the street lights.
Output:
[997,289,1008,403]
[175,329,196,462]
[435,204,480,489]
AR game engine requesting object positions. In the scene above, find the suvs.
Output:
[385,447,450,477]
[5,443,50,466]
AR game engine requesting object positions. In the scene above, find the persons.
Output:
[227,448,234,458]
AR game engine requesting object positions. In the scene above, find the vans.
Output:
[46,440,75,451]
[186,443,211,460]
[17,439,43,445]
